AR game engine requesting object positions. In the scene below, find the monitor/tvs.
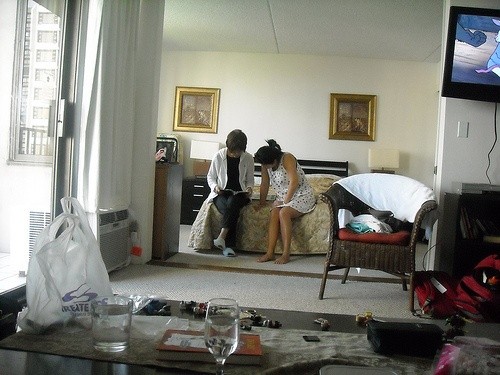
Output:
[441,7,500,103]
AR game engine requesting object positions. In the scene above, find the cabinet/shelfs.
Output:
[438,193,500,278]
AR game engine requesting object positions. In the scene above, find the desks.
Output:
[1,294,500,375]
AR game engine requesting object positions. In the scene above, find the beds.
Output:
[187,157,348,254]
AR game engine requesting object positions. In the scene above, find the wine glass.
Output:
[204,297,240,375]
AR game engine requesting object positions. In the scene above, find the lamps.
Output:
[190,140,219,177]
[368,148,399,174]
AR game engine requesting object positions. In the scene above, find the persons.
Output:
[254,139,319,264]
[206,129,254,257]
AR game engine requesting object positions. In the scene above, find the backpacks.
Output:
[456,254,500,323]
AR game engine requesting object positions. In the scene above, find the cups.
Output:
[91,296,133,354]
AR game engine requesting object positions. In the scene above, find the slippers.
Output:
[214,238,225,250]
[223,247,235,256]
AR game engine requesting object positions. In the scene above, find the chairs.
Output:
[319,173,437,312]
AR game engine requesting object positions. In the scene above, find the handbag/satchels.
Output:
[15,194,118,335]
[368,320,447,357]
[413,271,457,318]
[368,207,401,232]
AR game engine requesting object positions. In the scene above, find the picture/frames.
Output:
[329,93,377,141]
[173,86,220,134]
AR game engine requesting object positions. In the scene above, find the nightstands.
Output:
[180,176,211,224]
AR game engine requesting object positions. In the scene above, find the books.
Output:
[220,187,249,195]
